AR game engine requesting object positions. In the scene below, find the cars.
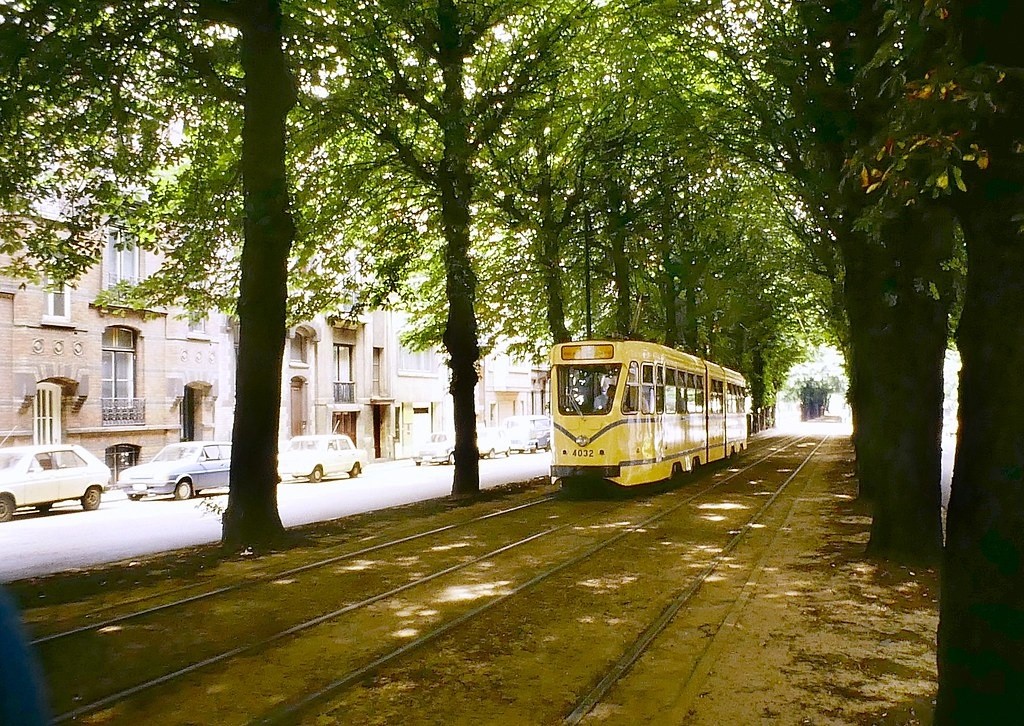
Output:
[115,442,231,501]
[279,435,368,485]
[477,427,511,459]
[411,433,456,467]
[0,444,113,522]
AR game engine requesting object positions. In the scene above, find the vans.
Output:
[504,415,550,455]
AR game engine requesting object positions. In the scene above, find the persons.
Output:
[606,384,615,397]
[625,387,649,411]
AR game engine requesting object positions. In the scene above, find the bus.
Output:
[548,337,746,491]
